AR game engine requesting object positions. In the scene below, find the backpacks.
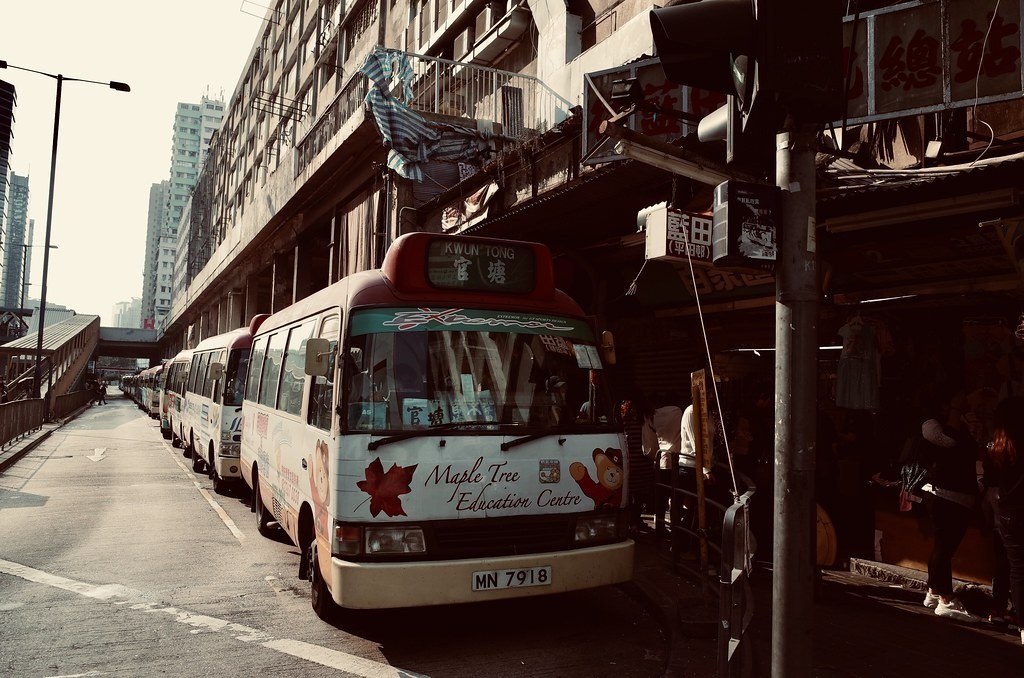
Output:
[899,433,929,515]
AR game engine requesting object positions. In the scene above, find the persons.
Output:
[640,385,683,540]
[98,384,108,405]
[746,384,775,465]
[0,375,9,404]
[679,380,716,529]
[915,381,983,625]
[978,394,1024,646]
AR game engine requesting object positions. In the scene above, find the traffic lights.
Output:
[649,0,769,140]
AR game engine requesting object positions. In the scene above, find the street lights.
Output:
[0,59,130,400]
[0,241,59,339]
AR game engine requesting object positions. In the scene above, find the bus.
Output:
[118,313,274,495]
[240,230,640,631]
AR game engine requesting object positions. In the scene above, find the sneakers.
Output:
[975,605,1005,621]
[1001,614,1024,632]
[922,591,940,608]
[934,597,982,623]
